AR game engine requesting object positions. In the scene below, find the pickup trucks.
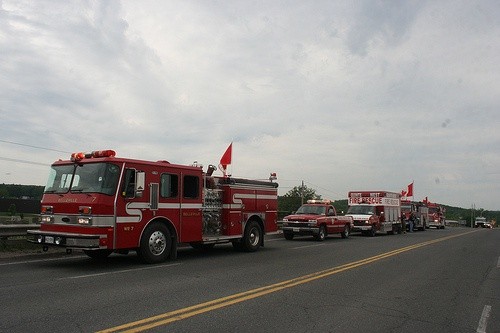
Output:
[282,199,353,241]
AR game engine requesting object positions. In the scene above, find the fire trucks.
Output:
[401,200,426,233]
[27,150,279,265]
[345,191,403,237]
[426,202,446,229]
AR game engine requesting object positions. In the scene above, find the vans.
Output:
[475,217,486,228]
